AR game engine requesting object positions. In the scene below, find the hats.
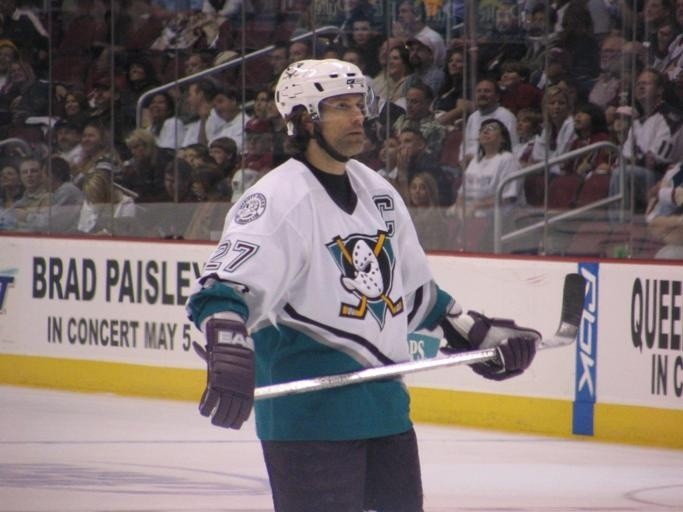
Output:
[244,118,274,132]
[231,168,261,203]
[614,106,638,117]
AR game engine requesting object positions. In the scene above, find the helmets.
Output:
[275,59,367,137]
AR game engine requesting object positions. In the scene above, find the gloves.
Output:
[440,310,541,381]
[192,318,254,430]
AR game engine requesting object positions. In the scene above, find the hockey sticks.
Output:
[254,273,586,401]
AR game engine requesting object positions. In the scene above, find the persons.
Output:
[183,56,543,511]
[1,1,682,265]
